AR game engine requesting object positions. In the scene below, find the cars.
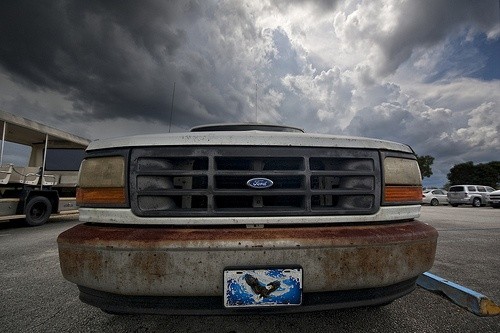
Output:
[421,188,449,205]
[55,123,439,318]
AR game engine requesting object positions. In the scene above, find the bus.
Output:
[0,112,90,226]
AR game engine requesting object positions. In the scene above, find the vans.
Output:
[448,184,495,206]
